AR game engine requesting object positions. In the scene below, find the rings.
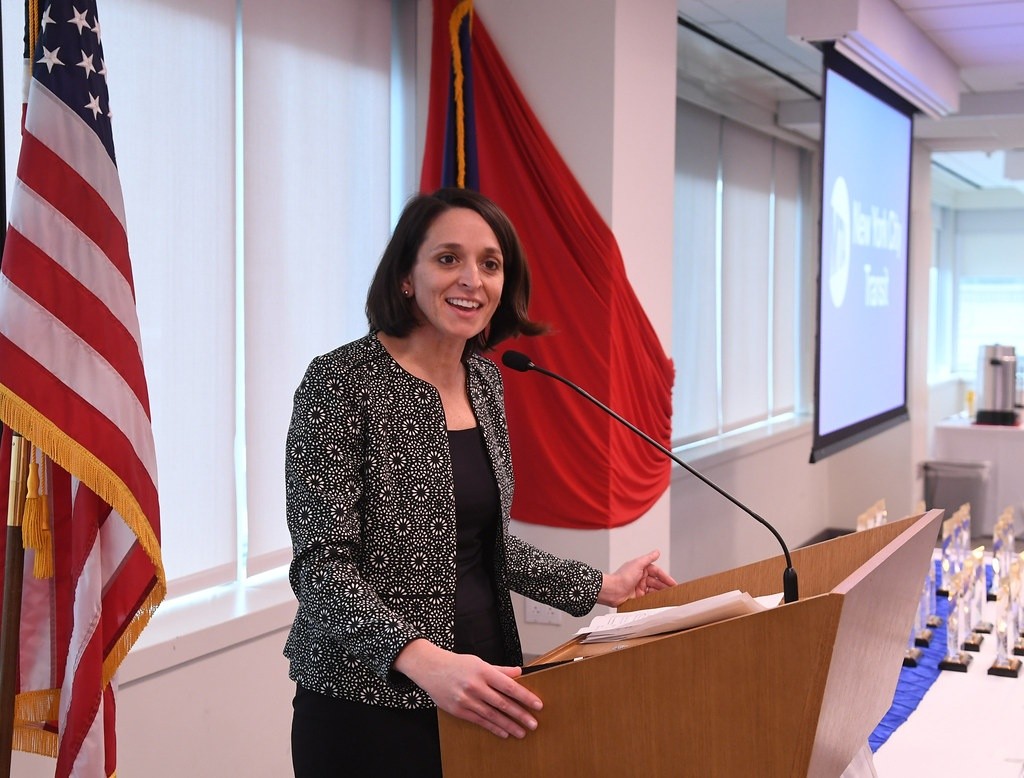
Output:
[646,587,649,593]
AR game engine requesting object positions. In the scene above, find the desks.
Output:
[934,405,1024,538]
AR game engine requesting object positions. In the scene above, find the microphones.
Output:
[502,350,799,603]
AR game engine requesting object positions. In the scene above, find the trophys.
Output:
[850,497,1023,678]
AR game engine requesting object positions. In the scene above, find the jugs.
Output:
[984,344,1015,411]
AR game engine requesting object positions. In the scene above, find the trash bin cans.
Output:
[925,459,997,540]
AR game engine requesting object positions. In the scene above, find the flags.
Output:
[0,0,167,778]
[422,3,672,529]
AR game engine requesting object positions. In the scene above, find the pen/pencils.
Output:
[522,655,595,675]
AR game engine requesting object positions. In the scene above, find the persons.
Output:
[283,188,678,778]
